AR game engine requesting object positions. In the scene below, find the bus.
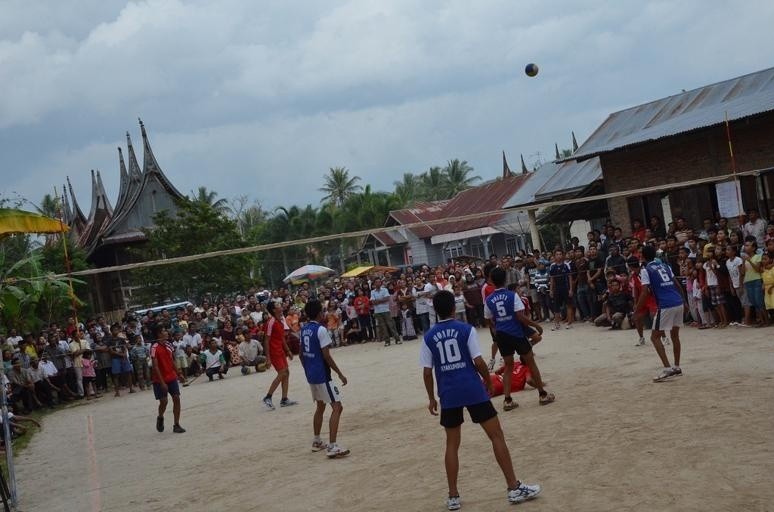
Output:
[130,301,200,317]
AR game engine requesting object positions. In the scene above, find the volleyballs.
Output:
[525,63,538,76]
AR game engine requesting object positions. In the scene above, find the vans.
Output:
[384,264,430,278]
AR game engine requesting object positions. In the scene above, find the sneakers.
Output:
[279,399,296,408]
[497,356,505,368]
[444,492,462,511]
[507,480,541,505]
[157,416,165,433]
[652,367,683,383]
[502,399,517,411]
[685,319,773,332]
[325,442,351,459]
[487,357,495,370]
[263,397,275,409]
[538,393,556,406]
[535,315,597,332]
[311,439,327,453]
[173,424,184,433]
[662,337,672,345]
[634,336,647,347]
[383,338,403,346]
